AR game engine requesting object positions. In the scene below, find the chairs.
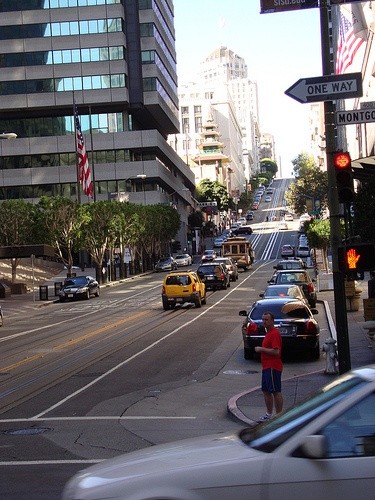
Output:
[281,276,289,283]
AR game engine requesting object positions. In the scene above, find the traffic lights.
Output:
[334,151,354,204]
[340,243,375,273]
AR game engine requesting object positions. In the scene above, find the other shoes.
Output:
[257,414,271,422]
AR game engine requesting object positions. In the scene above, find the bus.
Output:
[201,250,216,262]
[221,240,255,271]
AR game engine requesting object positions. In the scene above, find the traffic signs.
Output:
[285,73,362,104]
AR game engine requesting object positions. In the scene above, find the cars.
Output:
[175,253,192,267]
[258,285,311,311]
[266,269,318,308]
[284,214,293,221]
[279,223,288,230]
[154,256,178,273]
[281,245,295,257]
[61,364,375,500]
[240,296,322,361]
[272,234,309,274]
[60,275,101,302]
[297,212,310,232]
[213,176,276,248]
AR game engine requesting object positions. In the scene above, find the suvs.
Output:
[233,227,253,236]
[197,262,231,290]
[212,256,239,282]
[160,270,209,311]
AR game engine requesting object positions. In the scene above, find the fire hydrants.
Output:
[323,336,340,377]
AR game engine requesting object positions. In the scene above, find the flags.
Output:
[74,104,94,200]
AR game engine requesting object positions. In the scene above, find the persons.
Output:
[254,312,284,424]
